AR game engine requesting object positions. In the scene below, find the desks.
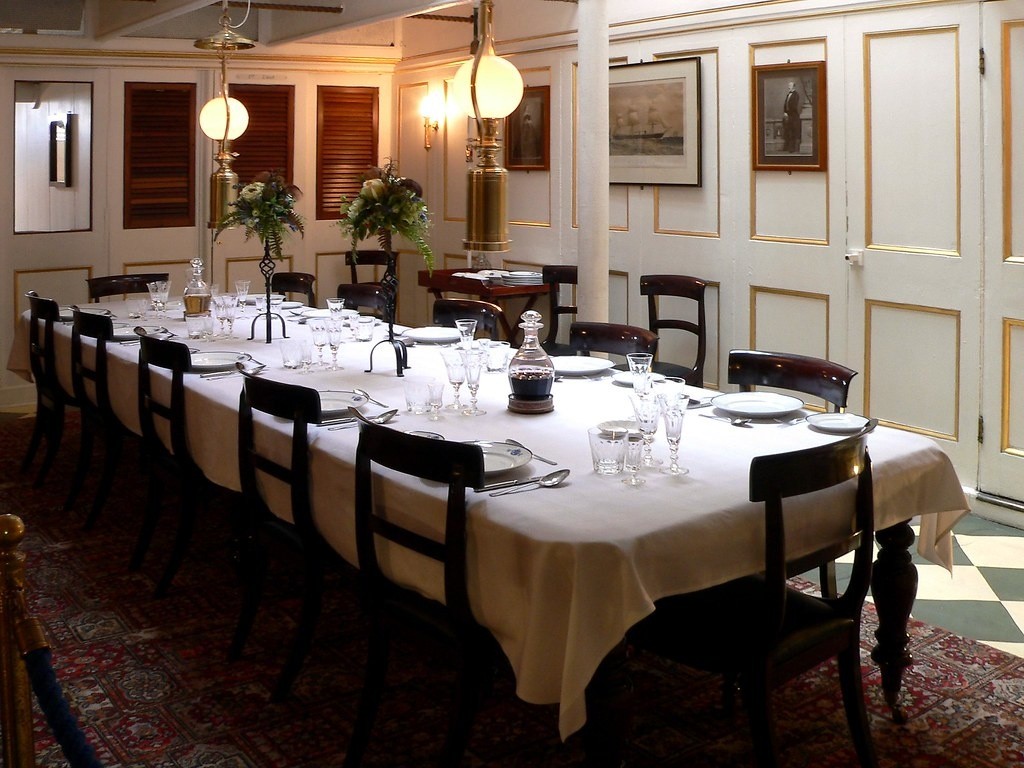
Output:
[7,293,971,768]
[418,269,559,349]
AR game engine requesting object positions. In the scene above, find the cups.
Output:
[186,313,203,340]
[126,298,147,319]
[587,427,628,475]
[487,340,511,373]
[402,375,431,413]
[356,316,374,341]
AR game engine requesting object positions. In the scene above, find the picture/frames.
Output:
[752,60,826,170]
[505,85,550,171]
[609,56,702,186]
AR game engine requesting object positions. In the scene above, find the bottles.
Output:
[508,310,555,414]
[183,258,212,321]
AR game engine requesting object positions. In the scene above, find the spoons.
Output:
[698,414,751,424]
[200,365,267,378]
[489,469,570,496]
[329,409,398,430]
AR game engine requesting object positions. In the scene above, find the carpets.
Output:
[0,410,1024,766]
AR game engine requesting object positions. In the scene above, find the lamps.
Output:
[193,0,256,229]
[421,97,440,149]
[452,0,523,252]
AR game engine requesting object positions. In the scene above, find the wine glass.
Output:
[429,319,487,421]
[622,352,688,484]
[204,281,265,343]
[146,280,171,320]
[299,297,360,373]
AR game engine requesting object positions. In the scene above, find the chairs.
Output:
[24,250,881,768]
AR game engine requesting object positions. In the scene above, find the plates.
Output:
[318,391,368,415]
[711,391,804,418]
[246,294,382,328]
[403,430,532,476]
[612,371,666,385]
[110,325,164,340]
[805,413,870,433]
[58,307,110,321]
[597,420,656,437]
[550,355,615,376]
[401,327,460,343]
[452,270,544,286]
[190,351,243,369]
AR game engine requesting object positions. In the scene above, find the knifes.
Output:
[473,476,545,491]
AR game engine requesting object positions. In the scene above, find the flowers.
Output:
[336,158,434,277]
[214,171,305,262]
